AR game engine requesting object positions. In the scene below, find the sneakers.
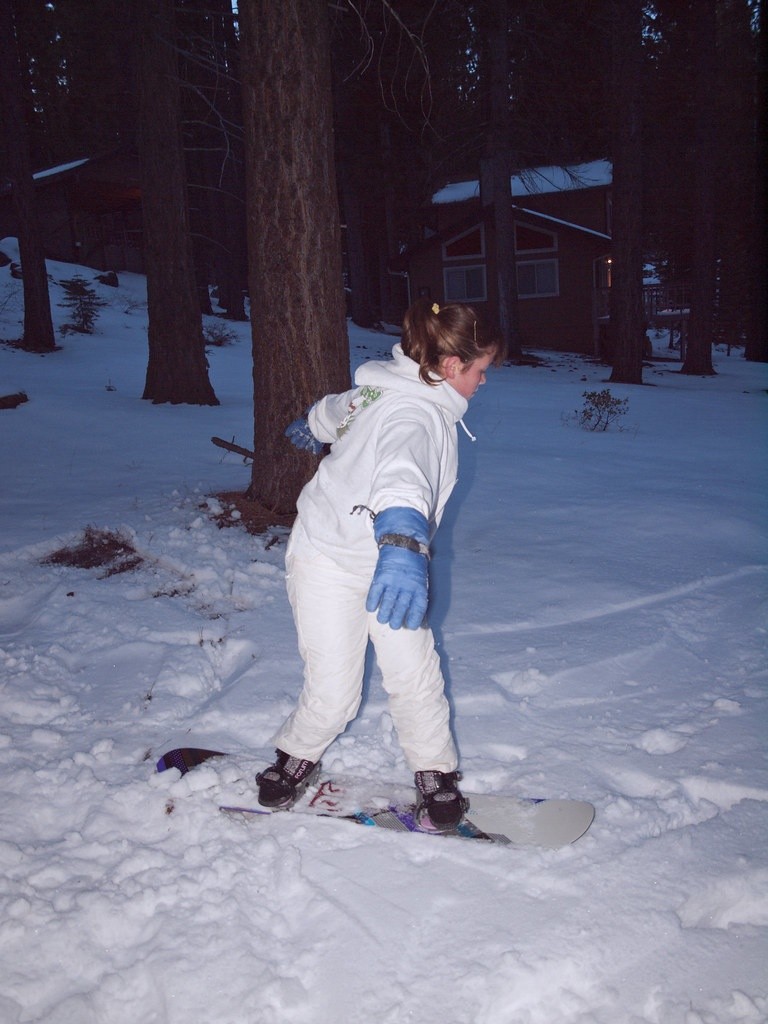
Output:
[256,751,313,806]
[415,768,465,831]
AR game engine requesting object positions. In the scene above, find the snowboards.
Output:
[153,746,598,853]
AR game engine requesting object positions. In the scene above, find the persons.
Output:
[254,298,503,829]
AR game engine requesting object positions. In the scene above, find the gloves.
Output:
[285,405,325,455]
[364,506,432,631]
